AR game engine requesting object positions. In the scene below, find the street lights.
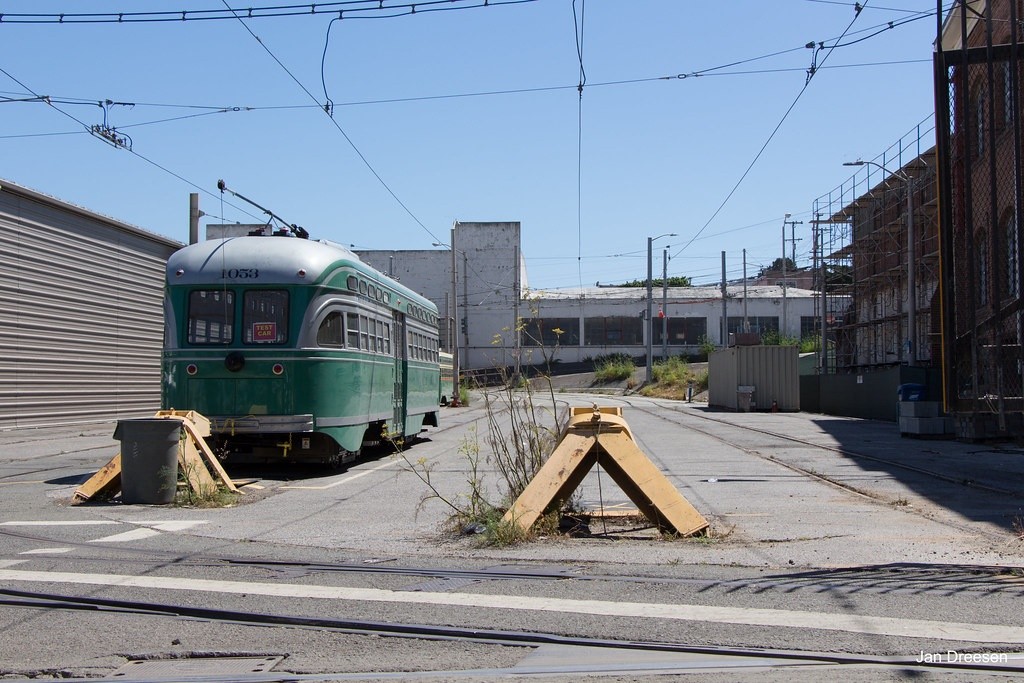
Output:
[782,212,793,339]
[432,242,469,389]
[646,232,680,384]
[843,160,915,368]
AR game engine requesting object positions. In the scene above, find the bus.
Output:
[160,178,442,481]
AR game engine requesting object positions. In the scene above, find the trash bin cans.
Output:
[113,417,187,504]
[897,384,922,401]
